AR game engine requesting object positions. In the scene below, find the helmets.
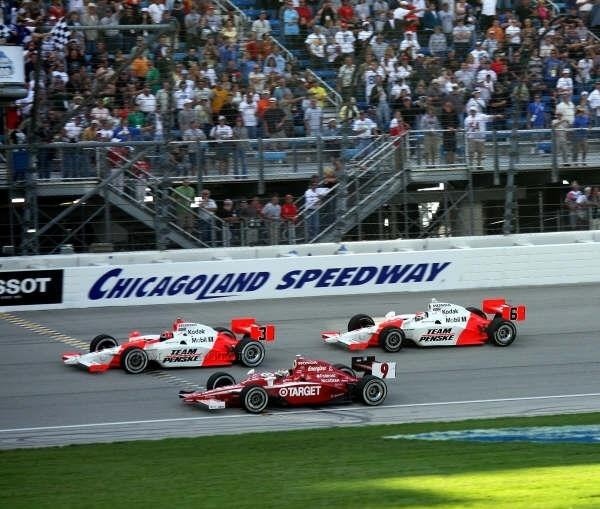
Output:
[416,311,426,321]
[275,368,290,378]
[160,330,172,339]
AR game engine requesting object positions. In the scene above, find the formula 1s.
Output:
[60,315,276,374]
[320,298,526,353]
[178,354,395,414]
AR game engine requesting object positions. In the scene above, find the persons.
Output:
[1,1,600,246]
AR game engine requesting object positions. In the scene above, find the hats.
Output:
[235,117,242,122]
[399,1,408,6]
[268,97,277,103]
[207,5,214,10]
[469,106,477,112]
[218,116,225,121]
[562,68,571,74]
